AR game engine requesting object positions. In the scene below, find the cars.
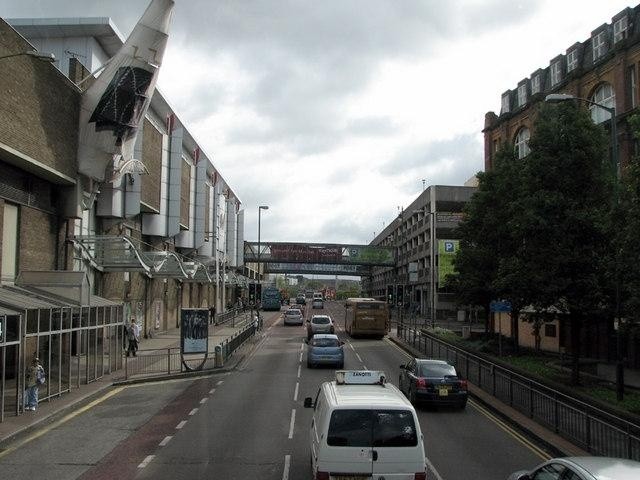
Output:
[305,314,334,340]
[398,358,469,413]
[508,456,639,480]
[305,334,345,368]
[283,292,324,326]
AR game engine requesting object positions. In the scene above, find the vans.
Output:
[303,370,428,480]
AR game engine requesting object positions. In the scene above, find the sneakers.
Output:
[30,408,35,411]
[25,407,29,410]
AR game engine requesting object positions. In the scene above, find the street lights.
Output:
[546,93,624,401]
[413,208,435,329]
[258,206,269,283]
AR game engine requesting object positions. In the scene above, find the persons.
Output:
[126,323,138,357]
[208,305,216,325]
[127,319,140,350]
[184,314,207,340]
[23,358,45,412]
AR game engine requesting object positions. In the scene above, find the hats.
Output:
[33,358,40,363]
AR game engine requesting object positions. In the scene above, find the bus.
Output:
[262,288,282,310]
[345,297,391,340]
